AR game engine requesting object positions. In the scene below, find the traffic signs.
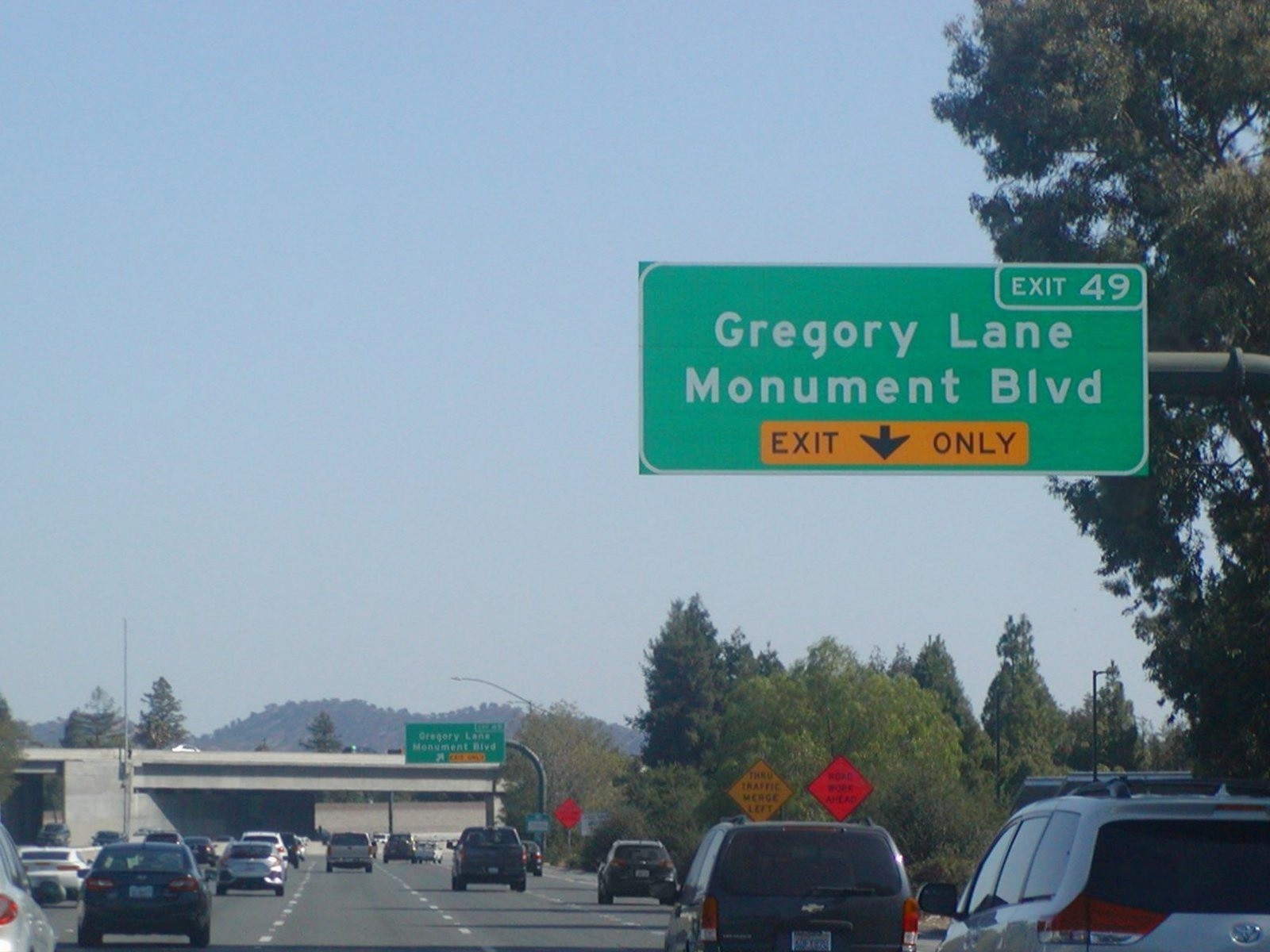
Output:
[637,256,1149,478]
[402,722,504,761]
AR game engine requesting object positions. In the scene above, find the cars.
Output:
[77,840,219,949]
[19,822,447,884]
[18,846,95,907]
[0,825,56,952]
[171,744,201,753]
[217,841,287,897]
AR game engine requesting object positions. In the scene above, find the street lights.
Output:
[452,676,533,715]
[1092,666,1116,783]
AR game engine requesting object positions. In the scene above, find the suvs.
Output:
[649,808,919,951]
[916,774,1269,952]
[520,840,542,876]
[445,825,525,891]
[594,837,683,907]
